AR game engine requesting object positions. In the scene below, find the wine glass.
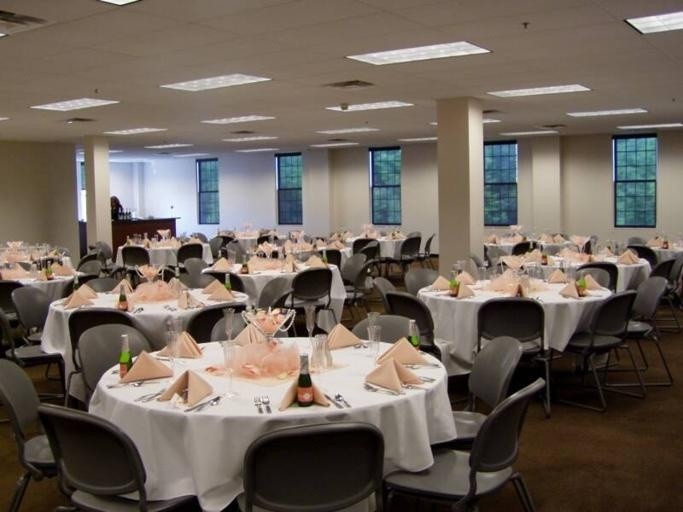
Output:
[219,339,239,401]
[222,307,236,343]
[303,302,383,396]
[164,315,185,380]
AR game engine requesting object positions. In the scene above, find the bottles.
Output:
[119,334,131,380]
[541,250,548,267]
[114,248,331,315]
[407,319,420,350]
[118,206,132,220]
[45,260,54,281]
[575,270,588,296]
[448,269,460,297]
[296,353,313,407]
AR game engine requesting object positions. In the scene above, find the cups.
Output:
[1,243,52,262]
[455,258,572,296]
[131,231,186,244]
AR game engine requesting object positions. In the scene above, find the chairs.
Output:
[33,402,201,511]
[232,418,391,510]
[0,359,81,510]
[380,376,547,510]
[1,223,682,420]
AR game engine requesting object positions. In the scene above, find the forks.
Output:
[254,397,265,417]
[262,394,269,413]
